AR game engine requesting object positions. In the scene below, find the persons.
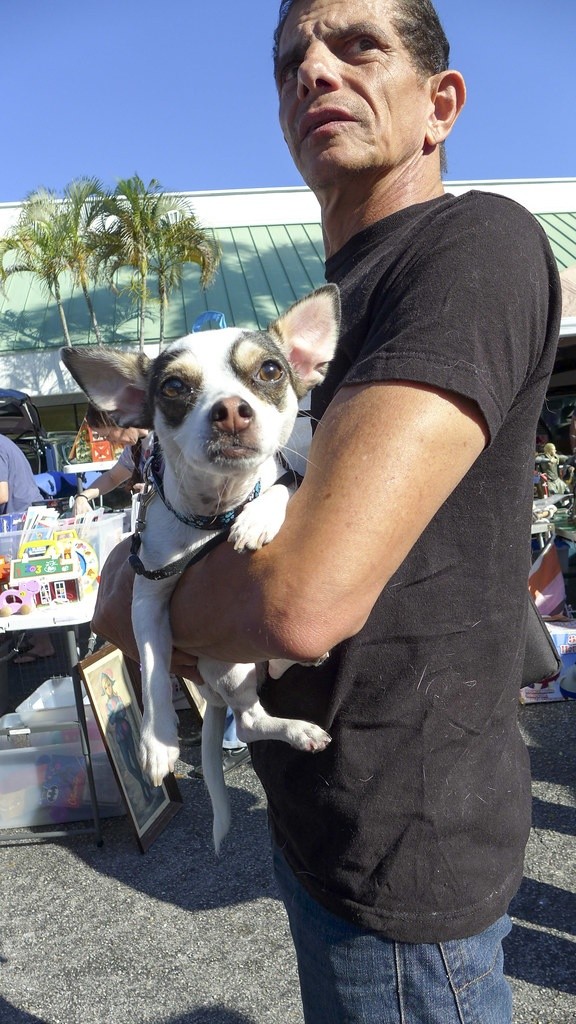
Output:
[66,401,158,518]
[178,689,253,781]
[0,431,57,665]
[88,0,561,1024]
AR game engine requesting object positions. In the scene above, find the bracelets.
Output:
[68,492,88,509]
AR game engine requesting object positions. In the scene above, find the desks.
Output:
[63,460,120,495]
[0,587,107,851]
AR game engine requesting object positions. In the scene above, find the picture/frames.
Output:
[75,642,186,855]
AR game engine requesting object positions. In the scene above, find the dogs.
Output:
[56,283,341,856]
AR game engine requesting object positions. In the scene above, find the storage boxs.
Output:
[0,705,131,832]
[15,674,96,729]
[0,511,128,596]
